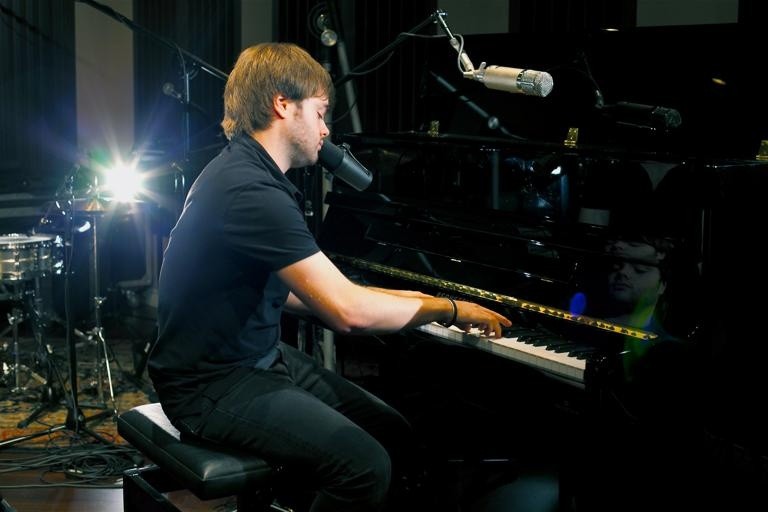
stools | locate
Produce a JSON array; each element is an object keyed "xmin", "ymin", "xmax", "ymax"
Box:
[{"xmin": 116, "ymin": 399, "xmax": 293, "ymax": 512}]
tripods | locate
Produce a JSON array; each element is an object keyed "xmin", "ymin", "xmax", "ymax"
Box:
[
  {"xmin": 0, "ymin": 215, "xmax": 118, "ymax": 448},
  {"xmin": 0, "ymin": 279, "xmax": 97, "ymax": 347},
  {"xmin": 13, "ymin": 343, "xmax": 107, "ymax": 429}
]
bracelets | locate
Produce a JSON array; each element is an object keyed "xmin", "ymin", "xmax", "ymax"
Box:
[{"xmin": 440, "ymin": 293, "xmax": 458, "ymax": 332}]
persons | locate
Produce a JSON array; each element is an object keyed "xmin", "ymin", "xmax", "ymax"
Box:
[
  {"xmin": 581, "ymin": 230, "xmax": 671, "ymax": 337},
  {"xmin": 145, "ymin": 38, "xmax": 513, "ymax": 510}
]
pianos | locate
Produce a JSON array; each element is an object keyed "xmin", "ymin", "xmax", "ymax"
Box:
[{"xmin": 307, "ymin": 23, "xmax": 761, "ymax": 510}]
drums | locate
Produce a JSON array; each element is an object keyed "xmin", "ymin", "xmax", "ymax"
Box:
[{"xmin": 1, "ymin": 233, "xmax": 63, "ymax": 283}]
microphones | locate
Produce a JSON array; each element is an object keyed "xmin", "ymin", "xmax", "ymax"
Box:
[
  {"xmin": 467, "ymin": 62, "xmax": 554, "ymax": 98},
  {"xmin": 162, "ymin": 83, "xmax": 210, "ymax": 119},
  {"xmin": 599, "ymin": 101, "xmax": 683, "ymax": 131},
  {"xmin": 318, "ymin": 140, "xmax": 374, "ymax": 193}
]
